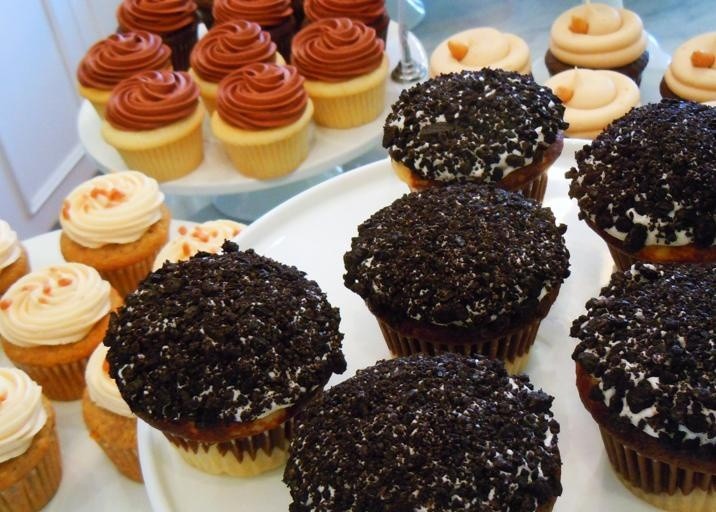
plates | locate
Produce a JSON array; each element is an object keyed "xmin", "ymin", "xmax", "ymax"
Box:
[
  {"xmin": 75, "ymin": 16, "xmax": 430, "ymax": 197},
  {"xmin": 136, "ymin": 138, "xmax": 667, "ymax": 512},
  {"xmin": 1, "ymin": 216, "xmax": 204, "ymax": 512}
]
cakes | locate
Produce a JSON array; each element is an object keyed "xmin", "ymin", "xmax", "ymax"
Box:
[
  {"xmin": 213, "ymin": 63, "xmax": 315, "ymax": 182},
  {"xmin": 284, "ymin": 351, "xmax": 561, "ymax": 512},
  {"xmin": 1, "ymin": 263, "xmax": 125, "ymax": 399},
  {"xmin": 116, "ymin": 0, "xmax": 208, "ymax": 69},
  {"xmin": 576, "ymin": 259, "xmax": 715, "ymax": 512},
  {"xmin": 344, "ymin": 183, "xmax": 572, "ymax": 374},
  {"xmin": 210, "ymin": 0, "xmax": 294, "ymax": 50},
  {"xmin": 1, "ymin": 219, "xmax": 32, "ymax": 295},
  {"xmin": 0, "ymin": 368, "xmax": 62, "ymax": 511},
  {"xmin": 153, "ymin": 219, "xmax": 246, "ymax": 275},
  {"xmin": 80, "ymin": 336, "xmax": 142, "ymax": 483},
  {"xmin": 544, "ymin": 69, "xmax": 639, "ymax": 139},
  {"xmin": 569, "ymin": 99, "xmax": 715, "ymax": 269},
  {"xmin": 190, "ymin": 19, "xmax": 285, "ymax": 116},
  {"xmin": 291, "ymin": 16, "xmax": 387, "ymax": 128},
  {"xmin": 77, "ymin": 29, "xmax": 174, "ymax": 120},
  {"xmin": 432, "ymin": 26, "xmax": 531, "ymax": 76},
  {"xmin": 108, "ymin": 246, "xmax": 340, "ymax": 475},
  {"xmin": 60, "ymin": 169, "xmax": 171, "ymax": 298},
  {"xmin": 102, "ymin": 70, "xmax": 203, "ymax": 180},
  {"xmin": 300, "ymin": 0, "xmax": 388, "ymax": 46},
  {"xmin": 548, "ymin": 6, "xmax": 650, "ymax": 82},
  {"xmin": 660, "ymin": 29, "xmax": 716, "ymax": 105},
  {"xmin": 383, "ymin": 69, "xmax": 566, "ymax": 209}
]
